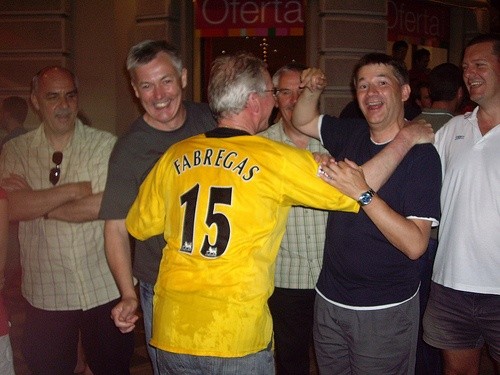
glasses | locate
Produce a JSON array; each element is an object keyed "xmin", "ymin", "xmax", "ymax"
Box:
[
  {"xmin": 259, "ymin": 85, "xmax": 280, "ymax": 97},
  {"xmin": 50, "ymin": 152, "xmax": 63, "ymax": 186}
]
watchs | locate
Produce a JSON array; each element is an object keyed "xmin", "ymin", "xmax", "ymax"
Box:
[{"xmin": 357, "ymin": 189, "xmax": 376, "ymax": 206}]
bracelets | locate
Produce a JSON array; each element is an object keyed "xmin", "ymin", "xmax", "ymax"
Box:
[{"xmin": 44, "ymin": 213, "xmax": 49, "ymax": 220}]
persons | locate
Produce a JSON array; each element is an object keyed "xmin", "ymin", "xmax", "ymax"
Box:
[{"xmin": 0, "ymin": 31, "xmax": 500, "ymax": 375}]
